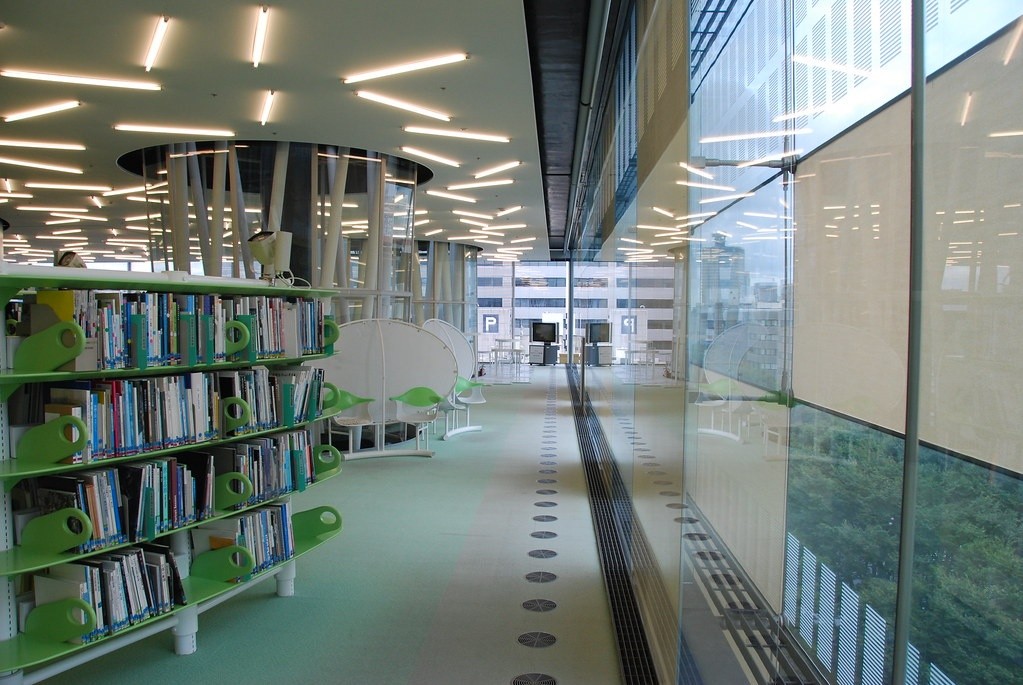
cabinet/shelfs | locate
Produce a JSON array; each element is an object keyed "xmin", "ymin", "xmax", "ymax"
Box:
[
  {"xmin": 585, "ymin": 345, "xmax": 612, "ymax": 367},
  {"xmin": 529, "ymin": 345, "xmax": 557, "ymax": 366},
  {"xmin": 0, "ymin": 262, "xmax": 343, "ymax": 685}
]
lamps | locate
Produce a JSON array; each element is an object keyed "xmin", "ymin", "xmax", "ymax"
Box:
[
  {"xmin": 339, "ymin": 50, "xmax": 471, "ymax": 85},
  {"xmin": 0, "ymin": 121, "xmax": 536, "ymax": 265},
  {"xmin": 58, "ymin": 252, "xmax": 87, "ymax": 268},
  {"xmin": 252, "ymin": 3, "xmax": 269, "ymax": 68},
  {"xmin": 248, "ymin": 230, "xmax": 295, "ymax": 286},
  {"xmin": 261, "ymin": 88, "xmax": 276, "ymax": 126},
  {"xmin": 144, "ymin": 10, "xmax": 171, "ymax": 72},
  {"xmin": 352, "ymin": 90, "xmax": 453, "ymax": 122},
  {"xmin": 0, "ymin": 65, "xmax": 163, "ymax": 91},
  {"xmin": 0, "ymin": 98, "xmax": 80, "ymax": 124}
]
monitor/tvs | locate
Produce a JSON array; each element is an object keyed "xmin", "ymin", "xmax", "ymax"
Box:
[
  {"xmin": 589, "ymin": 324, "xmax": 609, "ymax": 342},
  {"xmin": 532, "ymin": 322, "xmax": 556, "ymax": 342}
]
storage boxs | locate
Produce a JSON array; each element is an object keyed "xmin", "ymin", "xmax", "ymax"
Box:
[{"xmin": 31, "ymin": 304, "xmax": 98, "ymax": 372}]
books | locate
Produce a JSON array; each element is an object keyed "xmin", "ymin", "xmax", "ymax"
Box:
[
  {"xmin": 10, "ymin": 365, "xmax": 325, "ymax": 464},
  {"xmin": 15, "ymin": 428, "xmax": 329, "ymax": 554},
  {"xmin": 37, "ymin": 502, "xmax": 297, "ymax": 646},
  {"xmin": 36, "ymin": 286, "xmax": 325, "ymax": 373}
]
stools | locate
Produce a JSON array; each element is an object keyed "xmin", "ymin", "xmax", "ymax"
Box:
[{"xmin": 478, "ymin": 349, "xmax": 525, "ymax": 379}]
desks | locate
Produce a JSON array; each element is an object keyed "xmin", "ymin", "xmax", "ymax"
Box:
[
  {"xmin": 463, "ymin": 333, "xmax": 481, "ymax": 379},
  {"xmin": 492, "ymin": 339, "xmax": 525, "ymax": 379}
]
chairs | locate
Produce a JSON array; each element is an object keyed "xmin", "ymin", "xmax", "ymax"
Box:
[
  {"xmin": 325, "ymin": 390, "xmax": 375, "ymax": 454},
  {"xmin": 437, "ymin": 376, "xmax": 487, "ymax": 431},
  {"xmin": 389, "ymin": 386, "xmax": 445, "ymax": 451}
]
